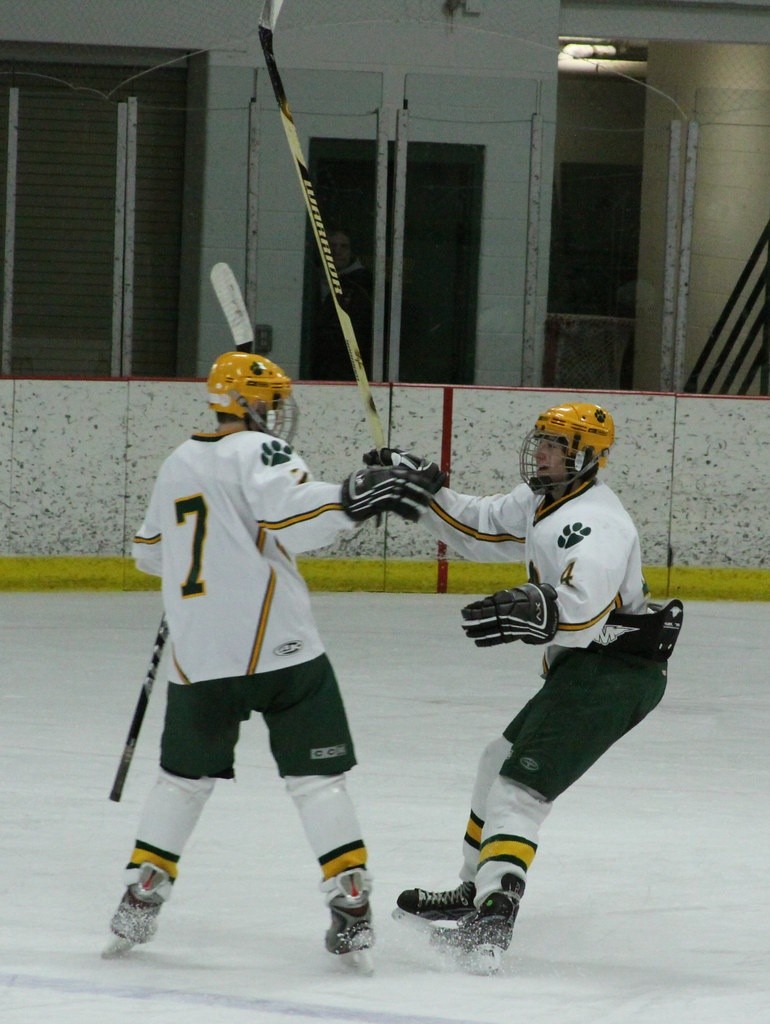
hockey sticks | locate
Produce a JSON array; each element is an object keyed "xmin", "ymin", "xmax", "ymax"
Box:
[
  {"xmin": 106, "ymin": 257, "xmax": 259, "ymax": 808},
  {"xmin": 255, "ymin": 0, "xmax": 395, "ymax": 469}
]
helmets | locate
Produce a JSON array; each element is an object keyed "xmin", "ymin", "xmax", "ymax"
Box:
[
  {"xmin": 519, "ymin": 403, "xmax": 615, "ymax": 495},
  {"xmin": 207, "ymin": 351, "xmax": 298, "ymax": 445}
]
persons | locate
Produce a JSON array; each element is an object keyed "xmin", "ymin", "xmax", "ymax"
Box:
[
  {"xmin": 312, "ymin": 225, "xmax": 373, "ymax": 382},
  {"xmin": 100, "ymin": 351, "xmax": 434, "ymax": 977},
  {"xmin": 363, "ymin": 403, "xmax": 668, "ymax": 973}
]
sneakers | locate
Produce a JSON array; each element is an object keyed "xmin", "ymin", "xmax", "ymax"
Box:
[
  {"xmin": 442, "ymin": 873, "xmax": 526, "ymax": 977},
  {"xmin": 391, "ymin": 881, "xmax": 479, "ymax": 952},
  {"xmin": 320, "ymin": 868, "xmax": 376, "ymax": 975},
  {"xmin": 102, "ymin": 862, "xmax": 169, "ymax": 960}
]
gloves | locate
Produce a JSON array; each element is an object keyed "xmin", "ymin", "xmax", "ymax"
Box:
[
  {"xmin": 342, "ymin": 466, "xmax": 433, "ymax": 524},
  {"xmin": 363, "ymin": 448, "xmax": 449, "ymax": 496},
  {"xmin": 461, "ymin": 584, "xmax": 559, "ymax": 648}
]
